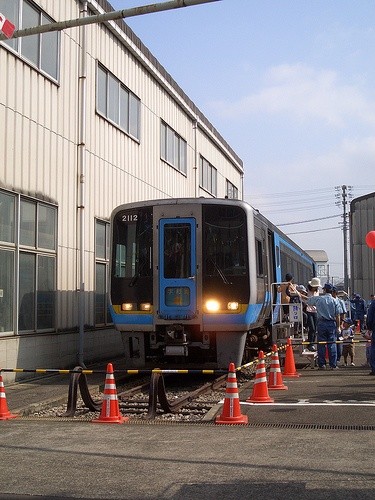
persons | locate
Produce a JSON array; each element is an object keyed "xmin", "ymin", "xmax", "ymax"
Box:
[
  {"xmin": 332, "ymin": 287, "xmax": 347, "ymax": 362},
  {"xmin": 305, "ymin": 278, "xmax": 322, "ymax": 350},
  {"xmin": 341, "ymin": 318, "xmax": 355, "ymax": 366},
  {"xmin": 350, "ymin": 294, "xmax": 367, "ymax": 331},
  {"xmin": 276, "ymin": 272, "xmax": 307, "ymax": 327},
  {"xmin": 368, "ymin": 294, "xmax": 375, "ymax": 305},
  {"xmin": 301, "ymin": 283, "xmax": 341, "ymax": 369},
  {"xmin": 365, "ymin": 300, "xmax": 375, "ymax": 375}
]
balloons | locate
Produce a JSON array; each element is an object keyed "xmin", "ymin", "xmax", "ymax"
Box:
[{"xmin": 366, "ymin": 231, "xmax": 375, "ymax": 248}]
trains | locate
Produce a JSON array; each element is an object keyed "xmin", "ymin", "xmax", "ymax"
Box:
[{"xmin": 108, "ymin": 196, "xmax": 316, "ymax": 369}]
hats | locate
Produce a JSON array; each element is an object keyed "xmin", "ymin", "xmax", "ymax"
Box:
[
  {"xmin": 322, "ymin": 283, "xmax": 333, "ymax": 290},
  {"xmin": 296, "ymin": 285, "xmax": 308, "ymax": 293},
  {"xmin": 341, "ymin": 318, "xmax": 353, "ymax": 324},
  {"xmin": 332, "ymin": 286, "xmax": 337, "ymax": 292},
  {"xmin": 356, "ymin": 295, "xmax": 361, "ymax": 299},
  {"xmin": 308, "ymin": 277, "xmax": 321, "ymax": 287},
  {"xmin": 286, "ymin": 273, "xmax": 294, "ymax": 282}
]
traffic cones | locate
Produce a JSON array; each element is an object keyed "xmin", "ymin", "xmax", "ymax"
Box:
[
  {"xmin": 215, "ymin": 363, "xmax": 248, "ymax": 424},
  {"xmin": 325, "ymin": 346, "xmax": 329, "ymax": 363},
  {"xmin": 267, "ymin": 345, "xmax": 288, "ymax": 390},
  {"xmin": 0, "ymin": 376, "xmax": 16, "ymax": 420},
  {"xmin": 281, "ymin": 338, "xmax": 301, "ymax": 377},
  {"xmin": 245, "ymin": 350, "xmax": 274, "ymax": 403},
  {"xmin": 355, "ymin": 320, "xmax": 360, "ymax": 333},
  {"xmin": 91, "ymin": 363, "xmax": 128, "ymax": 423}
]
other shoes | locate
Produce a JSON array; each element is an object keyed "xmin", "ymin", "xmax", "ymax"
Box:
[
  {"xmin": 351, "ymin": 362, "xmax": 355, "ymax": 366},
  {"xmin": 318, "ymin": 367, "xmax": 325, "ymax": 370},
  {"xmin": 306, "ymin": 345, "xmax": 316, "ymax": 351},
  {"xmin": 361, "ymin": 362, "xmax": 370, "ymax": 367},
  {"xmin": 294, "ymin": 334, "xmax": 302, "ymax": 338},
  {"xmin": 337, "ymin": 361, "xmax": 341, "ymax": 363},
  {"xmin": 342, "ymin": 363, "xmax": 348, "ymax": 366},
  {"xmin": 333, "ymin": 366, "xmax": 338, "ymax": 370},
  {"xmin": 369, "ymin": 371, "xmax": 375, "ymax": 376}
]
